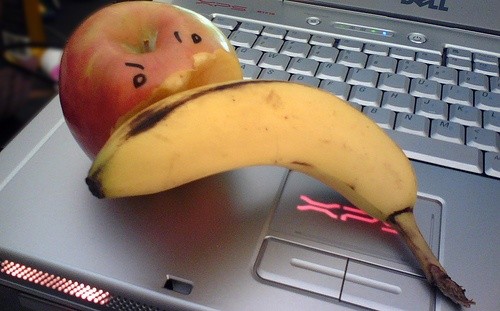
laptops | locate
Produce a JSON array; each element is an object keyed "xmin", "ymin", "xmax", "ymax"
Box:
[{"xmin": 1, "ymin": 0, "xmax": 500, "ymax": 309}]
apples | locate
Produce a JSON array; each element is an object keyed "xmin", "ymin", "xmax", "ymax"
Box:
[{"xmin": 59, "ymin": 0, "xmax": 243, "ymax": 163}]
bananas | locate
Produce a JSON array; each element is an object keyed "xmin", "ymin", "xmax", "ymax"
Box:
[{"xmin": 84, "ymin": 80, "xmax": 476, "ymax": 308}]
